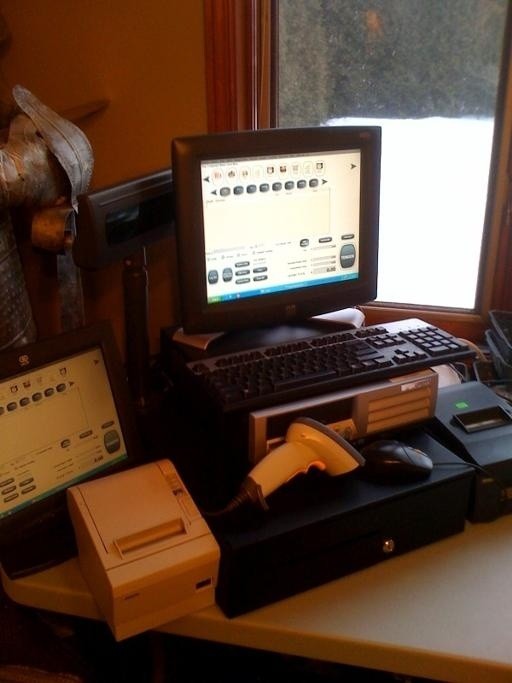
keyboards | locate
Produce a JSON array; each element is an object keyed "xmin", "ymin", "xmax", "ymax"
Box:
[{"xmin": 184, "ymin": 317, "xmax": 476, "ymax": 412}]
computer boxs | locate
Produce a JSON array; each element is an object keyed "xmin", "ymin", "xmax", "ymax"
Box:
[{"xmin": 159, "ymin": 320, "xmax": 439, "ymax": 471}]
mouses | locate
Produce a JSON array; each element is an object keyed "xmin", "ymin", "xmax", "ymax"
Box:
[{"xmin": 361, "ymin": 439, "xmax": 433, "ymax": 484}]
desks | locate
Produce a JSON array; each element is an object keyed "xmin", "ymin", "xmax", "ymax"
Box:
[{"xmin": 1, "ymin": 521, "xmax": 511, "ymax": 683}]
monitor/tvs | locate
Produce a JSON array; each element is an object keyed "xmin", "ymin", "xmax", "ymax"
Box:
[
  {"xmin": 172, "ymin": 127, "xmax": 381, "ymax": 350},
  {"xmin": 0, "ymin": 319, "xmax": 142, "ymax": 579}
]
